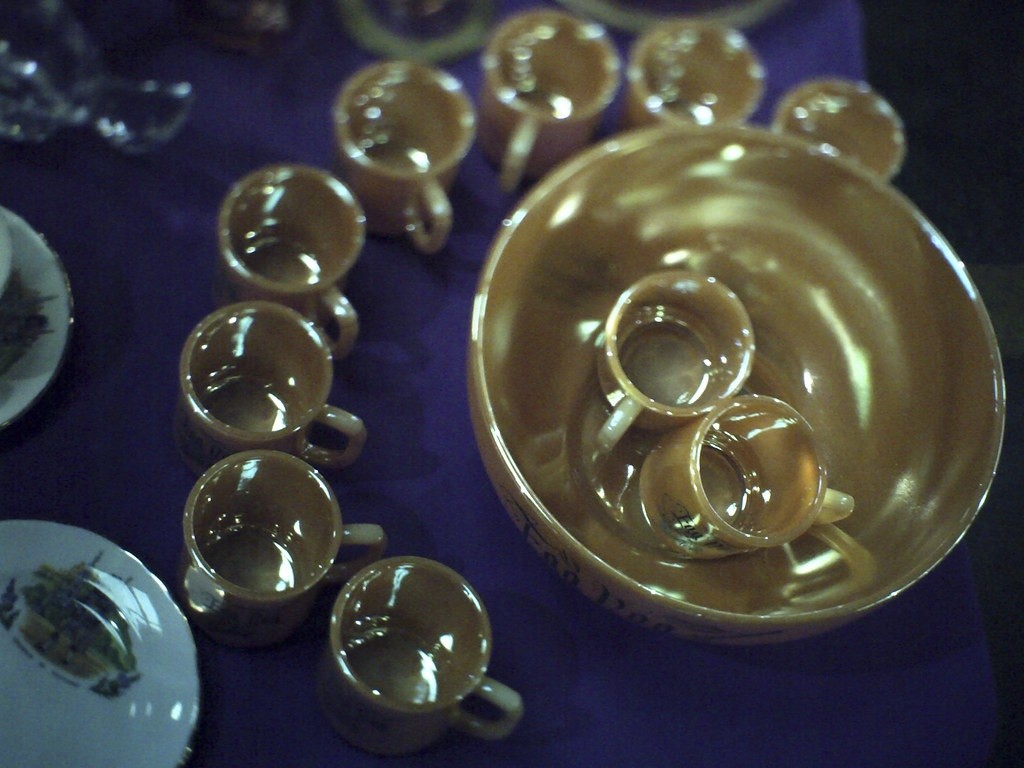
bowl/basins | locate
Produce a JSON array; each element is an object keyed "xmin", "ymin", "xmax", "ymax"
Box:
[{"xmin": 468, "ymin": 124, "xmax": 1008, "ymax": 636}]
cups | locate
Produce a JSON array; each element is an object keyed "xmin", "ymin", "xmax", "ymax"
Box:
[
  {"xmin": 772, "ymin": 78, "xmax": 908, "ymax": 187},
  {"xmin": 322, "ymin": 556, "xmax": 526, "ymax": 752},
  {"xmin": 202, "ymin": 162, "xmax": 368, "ymax": 357},
  {"xmin": 183, "ymin": 448, "xmax": 389, "ymax": 639},
  {"xmin": 329, "ymin": 61, "xmax": 482, "ymax": 253},
  {"xmin": 478, "ymin": 13, "xmax": 624, "ymax": 193},
  {"xmin": 176, "ymin": 298, "xmax": 370, "ymax": 475},
  {"xmin": 624, "ymin": 21, "xmax": 771, "ymax": 127},
  {"xmin": 593, "ymin": 272, "xmax": 757, "ymax": 454},
  {"xmin": 640, "ymin": 392, "xmax": 855, "ymax": 562}
]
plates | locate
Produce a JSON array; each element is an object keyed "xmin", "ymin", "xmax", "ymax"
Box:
[
  {"xmin": 338, "ymin": 1, "xmax": 498, "ymax": 63},
  {"xmin": 0, "ymin": 516, "xmax": 201, "ymax": 767},
  {"xmin": 0, "ymin": 208, "xmax": 71, "ymax": 428},
  {"xmin": 560, "ymin": 0, "xmax": 780, "ymax": 41}
]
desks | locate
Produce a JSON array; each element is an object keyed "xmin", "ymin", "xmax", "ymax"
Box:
[{"xmin": 0, "ymin": 0, "xmax": 999, "ymax": 768}]
